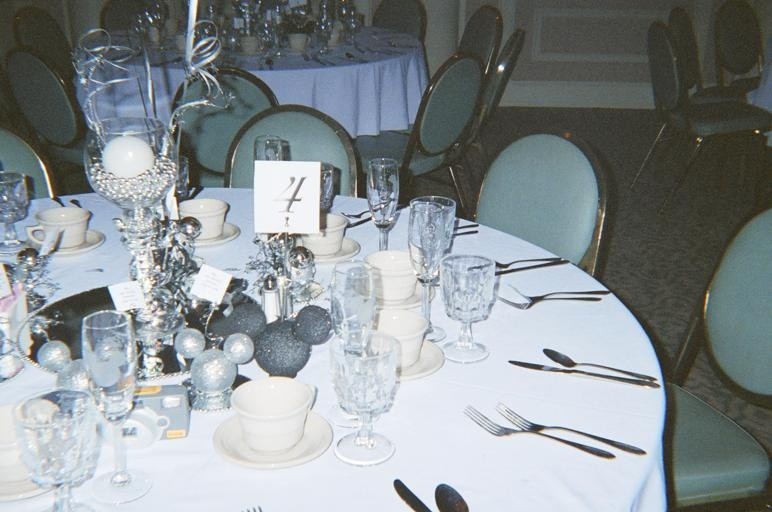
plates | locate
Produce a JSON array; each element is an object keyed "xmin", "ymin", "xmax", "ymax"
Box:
[
  {"xmin": 0, "ymin": 407, "xmax": 85, "ymax": 504},
  {"xmin": 33, "ymin": 230, "xmax": 104, "ymax": 257},
  {"xmin": 356, "ymin": 340, "xmax": 443, "ymax": 378},
  {"xmin": 314, "ymin": 235, "xmax": 361, "ymax": 260},
  {"xmin": 212, "ymin": 418, "xmax": 332, "ymax": 471},
  {"xmin": 191, "ymin": 223, "xmax": 242, "ymax": 249},
  {"xmin": 354, "ymin": 285, "xmax": 435, "ymax": 306}
]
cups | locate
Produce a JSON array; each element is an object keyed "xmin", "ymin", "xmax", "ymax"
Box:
[
  {"xmin": 299, "ymin": 213, "xmax": 349, "ymax": 254},
  {"xmin": 342, "ymin": 308, "xmax": 429, "ymax": 368},
  {"xmin": 251, "ymin": 136, "xmax": 292, "ymax": 160},
  {"xmin": 232, "ymin": 376, "xmax": 313, "ymax": 453},
  {"xmin": 176, "ymin": 199, "xmax": 230, "ymax": 239},
  {"xmin": 361, "ymin": 251, "xmax": 416, "ymax": 301},
  {"xmin": 25, "ymin": 209, "xmax": 89, "ymax": 247},
  {"xmin": 319, "ymin": 161, "xmax": 335, "ymax": 211}
]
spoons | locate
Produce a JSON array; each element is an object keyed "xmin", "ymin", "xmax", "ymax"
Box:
[
  {"xmin": 434, "ymin": 484, "xmax": 466, "ymax": 509},
  {"xmin": 543, "ymin": 345, "xmax": 654, "ymax": 384}
]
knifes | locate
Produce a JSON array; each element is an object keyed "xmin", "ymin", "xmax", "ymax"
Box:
[
  {"xmin": 507, "ymin": 357, "xmax": 660, "ymax": 393},
  {"xmin": 490, "ymin": 291, "xmax": 603, "ymax": 315},
  {"xmin": 392, "ymin": 477, "xmax": 434, "ymax": 510},
  {"xmin": 510, "ymin": 282, "xmax": 614, "ymax": 302}
]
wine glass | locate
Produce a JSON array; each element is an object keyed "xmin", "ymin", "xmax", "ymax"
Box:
[
  {"xmin": 411, "ymin": 196, "xmax": 458, "ymax": 303},
  {"xmin": 406, "ymin": 204, "xmax": 447, "ymax": 344},
  {"xmin": 367, "ymin": 158, "xmax": 401, "ymax": 252},
  {"xmin": 78, "ymin": 310, "xmax": 154, "ymax": 506},
  {"xmin": 18, "ymin": 388, "xmax": 107, "ymax": 510},
  {"xmin": 438, "ymin": 255, "xmax": 498, "ymax": 363},
  {"xmin": 120, "ymin": 1, "xmax": 381, "ymax": 64},
  {"xmin": 326, "ymin": 258, "xmax": 386, "ymax": 431},
  {"xmin": 1, "ymin": 170, "xmax": 30, "ymax": 253},
  {"xmin": 329, "ymin": 327, "xmax": 400, "ymax": 467}
]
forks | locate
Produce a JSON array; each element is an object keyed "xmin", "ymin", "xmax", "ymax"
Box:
[
  {"xmin": 460, "ymin": 405, "xmax": 621, "ymax": 460},
  {"xmin": 498, "ymin": 410, "xmax": 649, "ymax": 458}
]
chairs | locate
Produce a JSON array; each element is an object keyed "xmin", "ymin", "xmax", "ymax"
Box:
[
  {"xmin": 626, "ymin": 1, "xmax": 771, "ymax": 216},
  {"xmin": 351, "ymin": 0, "xmax": 526, "ymax": 221},
  {"xmin": 469, "ymin": 126, "xmax": 611, "ymax": 280},
  {"xmin": 99, "ymin": 0, "xmax": 169, "ymax": 36},
  {"xmin": 2, "ymin": 48, "xmax": 89, "ymax": 194},
  {"xmin": 663, "ymin": 201, "xmax": 772, "ymax": 512},
  {"xmin": 171, "ymin": 67, "xmax": 280, "ymax": 187},
  {"xmin": 0, "ymin": 118, "xmax": 58, "ymax": 201},
  {"xmin": 13, "ymin": 6, "xmax": 75, "ymax": 85},
  {"xmin": 223, "ymin": 104, "xmax": 364, "ymax": 198}
]
